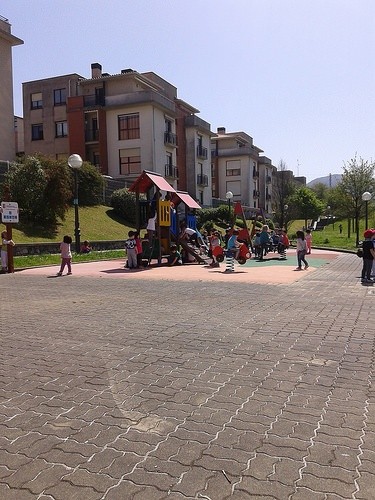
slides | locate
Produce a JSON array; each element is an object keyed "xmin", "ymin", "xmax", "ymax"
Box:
[{"xmin": 195, "ymin": 228, "xmax": 209, "ymax": 251}]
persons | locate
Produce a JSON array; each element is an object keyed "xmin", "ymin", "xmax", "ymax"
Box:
[
  {"xmin": 361, "ymin": 228, "xmax": 375, "ymax": 283},
  {"xmin": 292, "ymin": 231, "xmax": 309, "ymax": 271},
  {"xmin": 124, "ymin": 230, "xmax": 145, "ymax": 270},
  {"xmin": 146, "ymin": 209, "xmax": 158, "ymax": 252},
  {"xmin": 176, "ymin": 223, "xmax": 201, "ymax": 248},
  {"xmin": 227, "ymin": 229, "xmax": 244, "ymax": 260},
  {"xmin": 0, "ymin": 230, "xmax": 15, "ymax": 274},
  {"xmin": 253, "ymin": 225, "xmax": 290, "ymax": 256},
  {"xmin": 200, "ymin": 231, "xmax": 209, "ymax": 256},
  {"xmin": 305, "ymin": 230, "xmax": 312, "ymax": 254},
  {"xmin": 80, "ymin": 240, "xmax": 92, "ymax": 253},
  {"xmin": 167, "ymin": 244, "xmax": 182, "ymax": 266},
  {"xmin": 203, "ymin": 228, "xmax": 230, "ymax": 268},
  {"xmin": 150, "ymin": 192, "xmax": 176, "ymax": 220},
  {"xmin": 56, "ymin": 235, "xmax": 73, "ymax": 276}
]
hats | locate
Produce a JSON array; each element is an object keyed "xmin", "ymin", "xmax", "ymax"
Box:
[{"xmin": 364, "ymin": 229, "xmax": 375, "ymax": 237}]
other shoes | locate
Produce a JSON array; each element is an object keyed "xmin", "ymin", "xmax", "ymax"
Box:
[
  {"xmin": 199, "ymin": 247, "xmax": 204, "ymax": 253},
  {"xmin": 304, "ymin": 265, "xmax": 309, "ymax": 270},
  {"xmin": 129, "ymin": 267, "xmax": 132, "ymax": 270},
  {"xmin": 367, "ymin": 279, "xmax": 374, "ymax": 282},
  {"xmin": 56, "ymin": 273, "xmax": 62, "ymax": 276},
  {"xmin": 361, "ymin": 278, "xmax": 366, "ymax": 282},
  {"xmin": 67, "ymin": 272, "xmax": 72, "ymax": 275},
  {"xmin": 295, "ymin": 267, "xmax": 301, "ymax": 271},
  {"xmin": 134, "ymin": 267, "xmax": 138, "ymax": 269}
]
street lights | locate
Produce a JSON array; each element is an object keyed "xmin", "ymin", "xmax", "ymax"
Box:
[
  {"xmin": 67, "ymin": 154, "xmax": 84, "ymax": 254},
  {"xmin": 362, "ymin": 192, "xmax": 372, "ymax": 230},
  {"xmin": 226, "ymin": 191, "xmax": 234, "ymax": 214},
  {"xmin": 283, "ymin": 205, "xmax": 289, "ymax": 233},
  {"xmin": 326, "ymin": 205, "xmax": 331, "ymax": 226}
]
